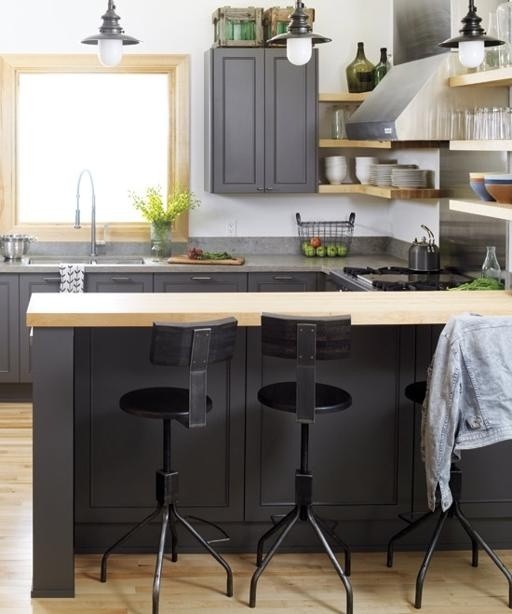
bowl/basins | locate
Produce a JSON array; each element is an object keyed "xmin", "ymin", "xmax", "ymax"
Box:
[
  {"xmin": 324, "ymin": 156, "xmax": 347, "ymax": 184},
  {"xmin": 0, "ymin": 234, "xmax": 32, "ymax": 259},
  {"xmin": 469, "ymin": 172, "xmax": 512, "ymax": 204},
  {"xmin": 354, "ymin": 157, "xmax": 378, "ymax": 184}
]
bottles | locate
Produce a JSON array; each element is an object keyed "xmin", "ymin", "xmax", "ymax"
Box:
[
  {"xmin": 345, "ymin": 42, "xmax": 392, "ymax": 92},
  {"xmin": 331, "ymin": 104, "xmax": 359, "ymax": 138},
  {"xmin": 476, "ymin": 2, "xmax": 512, "ymax": 72},
  {"xmin": 152, "ymin": 241, "xmax": 161, "ymax": 261},
  {"xmin": 481, "ymin": 246, "xmax": 501, "ymax": 283}
]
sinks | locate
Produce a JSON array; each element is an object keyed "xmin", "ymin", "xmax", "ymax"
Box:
[
  {"xmin": 93, "ymin": 253, "xmax": 147, "ymax": 268},
  {"xmin": 24, "ymin": 255, "xmax": 92, "ymax": 268}
]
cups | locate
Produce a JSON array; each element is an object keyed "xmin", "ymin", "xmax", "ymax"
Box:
[
  {"xmin": 450, "ymin": 111, "xmax": 463, "ymax": 140},
  {"xmin": 464, "ymin": 107, "xmax": 512, "ymax": 139}
]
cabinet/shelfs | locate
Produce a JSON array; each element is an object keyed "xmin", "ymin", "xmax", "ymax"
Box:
[
  {"xmin": 203, "ymin": 47, "xmax": 320, "ymax": 194},
  {"xmin": 448, "ymin": 66, "xmax": 512, "ymax": 221},
  {"xmin": 317, "ymin": 91, "xmax": 450, "ymax": 200},
  {"xmin": 153, "ymin": 272, "xmax": 322, "ymax": 292},
  {"xmin": 0, "ymin": 273, "xmax": 20, "ymax": 383},
  {"xmin": 20, "ymin": 273, "xmax": 153, "ymax": 383}
]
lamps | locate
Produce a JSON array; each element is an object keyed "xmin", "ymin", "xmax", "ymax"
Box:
[
  {"xmin": 438, "ymin": 0, "xmax": 505, "ymax": 69},
  {"xmin": 266, "ymin": 0, "xmax": 332, "ymax": 66},
  {"xmin": 81, "ymin": 0, "xmax": 139, "ymax": 68}
]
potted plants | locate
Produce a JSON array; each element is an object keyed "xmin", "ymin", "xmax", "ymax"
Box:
[{"xmin": 127, "ymin": 184, "xmax": 202, "ymax": 260}]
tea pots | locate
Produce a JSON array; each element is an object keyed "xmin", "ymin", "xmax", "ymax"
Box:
[{"xmin": 408, "ymin": 223, "xmax": 440, "ymax": 271}]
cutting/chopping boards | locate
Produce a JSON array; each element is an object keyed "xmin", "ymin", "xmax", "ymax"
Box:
[{"xmin": 168, "ymin": 254, "xmax": 244, "ymax": 265}]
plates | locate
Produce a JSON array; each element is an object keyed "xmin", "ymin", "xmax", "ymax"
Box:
[
  {"xmin": 391, "ymin": 169, "xmax": 427, "ymax": 188},
  {"xmin": 371, "ymin": 164, "xmax": 416, "ymax": 185}
]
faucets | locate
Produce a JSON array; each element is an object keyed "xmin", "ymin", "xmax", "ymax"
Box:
[{"xmin": 69, "ymin": 166, "xmax": 97, "ymax": 258}]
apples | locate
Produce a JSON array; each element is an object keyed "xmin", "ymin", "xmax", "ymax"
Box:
[{"xmin": 303, "ymin": 236, "xmax": 349, "ymax": 258}]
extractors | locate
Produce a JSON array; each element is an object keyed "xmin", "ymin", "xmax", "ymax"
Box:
[{"xmin": 344, "ymin": 51, "xmax": 469, "ymax": 141}]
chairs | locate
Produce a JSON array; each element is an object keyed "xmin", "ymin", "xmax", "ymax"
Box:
[
  {"xmin": 100, "ymin": 317, "xmax": 239, "ymax": 614},
  {"xmin": 248, "ymin": 313, "xmax": 354, "ymax": 613},
  {"xmin": 386, "ymin": 315, "xmax": 512, "ymax": 609}
]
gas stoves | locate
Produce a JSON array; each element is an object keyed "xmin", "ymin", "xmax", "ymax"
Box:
[{"xmin": 331, "ymin": 265, "xmax": 474, "ymax": 291}]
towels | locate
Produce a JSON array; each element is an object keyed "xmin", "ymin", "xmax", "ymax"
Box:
[{"xmin": 59, "ymin": 263, "xmax": 86, "ymax": 294}]
